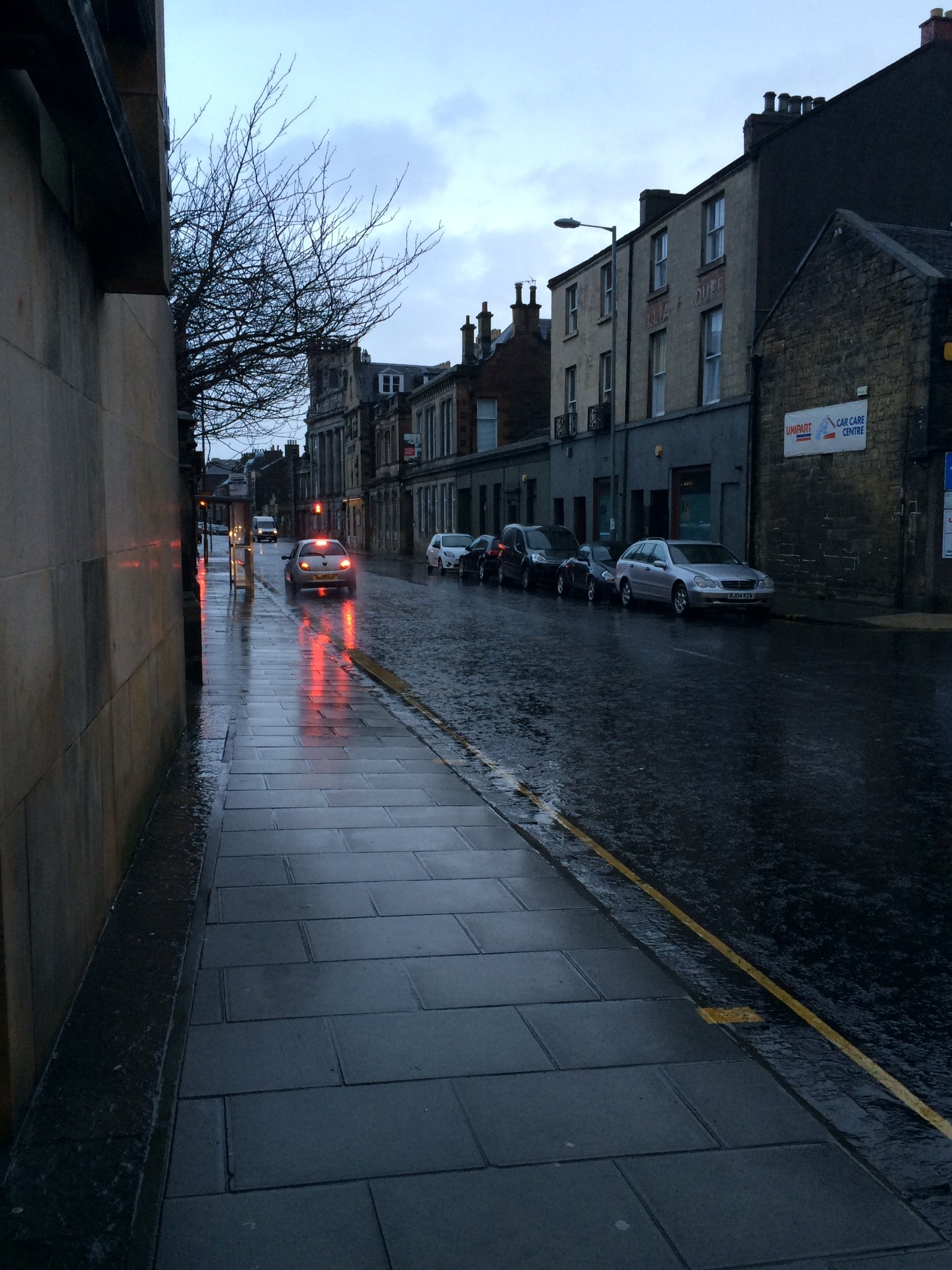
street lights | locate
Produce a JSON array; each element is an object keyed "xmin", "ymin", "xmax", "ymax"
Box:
[
  {"xmin": 199, "ymin": 501, "xmax": 208, "ymax": 563},
  {"xmin": 553, "ymin": 217, "xmax": 619, "ymax": 544}
]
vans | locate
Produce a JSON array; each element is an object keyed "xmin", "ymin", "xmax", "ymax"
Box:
[{"xmin": 252, "ymin": 516, "xmax": 278, "ymax": 543}]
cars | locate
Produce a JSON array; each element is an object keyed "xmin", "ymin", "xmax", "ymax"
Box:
[
  {"xmin": 427, "ymin": 522, "xmax": 776, "ymax": 616},
  {"xmin": 197, "ymin": 522, "xmax": 229, "ymax": 535},
  {"xmin": 282, "ymin": 539, "xmax": 357, "ymax": 593},
  {"xmin": 197, "ymin": 526, "xmax": 202, "ymax": 542}
]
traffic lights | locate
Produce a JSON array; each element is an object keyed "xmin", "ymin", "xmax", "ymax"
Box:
[{"xmin": 314, "ymin": 505, "xmax": 322, "ymax": 516}]
